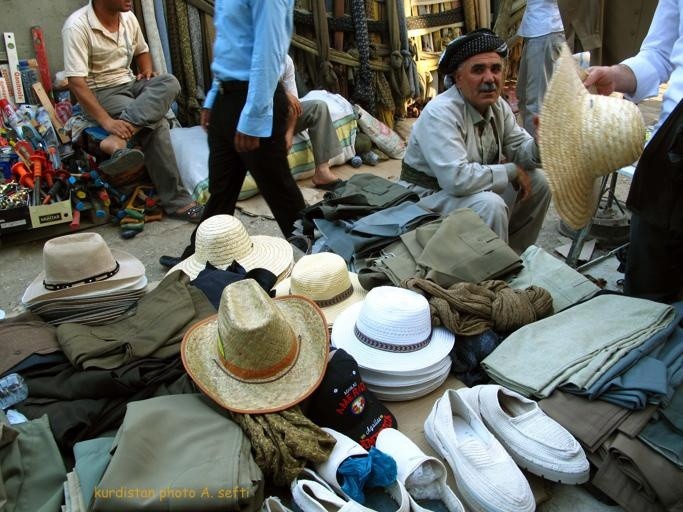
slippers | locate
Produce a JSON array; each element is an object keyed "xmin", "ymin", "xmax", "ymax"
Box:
[
  {"xmin": 316, "ymin": 178, "xmax": 343, "ymax": 190},
  {"xmin": 170, "ymin": 202, "xmax": 205, "ymax": 223},
  {"xmin": 98, "ymin": 149, "xmax": 144, "ymax": 175}
]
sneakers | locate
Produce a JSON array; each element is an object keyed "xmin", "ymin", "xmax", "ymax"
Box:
[{"xmin": 260, "ymin": 384, "xmax": 590, "ymax": 512}]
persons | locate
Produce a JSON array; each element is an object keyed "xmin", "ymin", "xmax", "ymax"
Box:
[
  {"xmin": 396, "ymin": 26, "xmax": 551, "ymax": 255},
  {"xmin": 158, "ymin": 1, "xmax": 307, "ymax": 270},
  {"xmin": 581, "ymin": 0, "xmax": 682, "ymax": 305},
  {"xmin": 492, "ymin": 1, "xmax": 602, "ymax": 144},
  {"xmin": 61, "ymin": 1, "xmax": 205, "ymax": 224},
  {"xmin": 278, "ymin": 53, "xmax": 344, "ymax": 190}
]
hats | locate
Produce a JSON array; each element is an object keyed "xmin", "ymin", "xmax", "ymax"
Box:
[
  {"xmin": 22, "ymin": 232, "xmax": 148, "ymax": 326},
  {"xmin": 438, "ymin": 28, "xmax": 507, "ymax": 74},
  {"xmin": 167, "ymin": 214, "xmax": 456, "ymax": 451},
  {"xmin": 538, "ymin": 42, "xmax": 646, "ymax": 230}
]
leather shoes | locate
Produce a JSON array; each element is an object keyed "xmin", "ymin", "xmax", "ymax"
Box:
[{"xmin": 160, "ymin": 256, "xmax": 180, "ymax": 267}]
